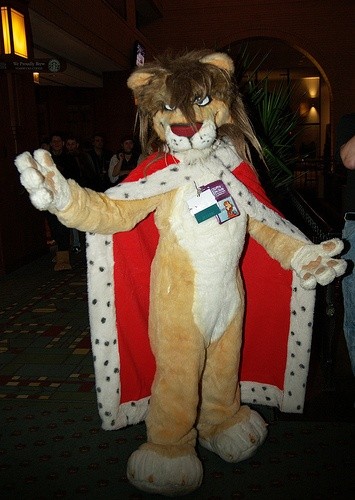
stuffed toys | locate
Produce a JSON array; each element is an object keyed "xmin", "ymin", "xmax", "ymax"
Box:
[{"xmin": 13, "ymin": 49, "xmax": 349, "ymax": 499}]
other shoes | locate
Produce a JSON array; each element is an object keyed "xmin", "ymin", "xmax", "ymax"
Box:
[
  {"xmin": 54, "ymin": 250, "xmax": 73, "ymax": 271},
  {"xmin": 72, "ymin": 246, "xmax": 82, "ymax": 257}
]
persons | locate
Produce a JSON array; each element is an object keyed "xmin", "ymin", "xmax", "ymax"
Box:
[
  {"xmin": 330, "ymin": 114, "xmax": 355, "ymax": 384},
  {"xmin": 29, "ymin": 132, "xmax": 141, "ymax": 273}
]
